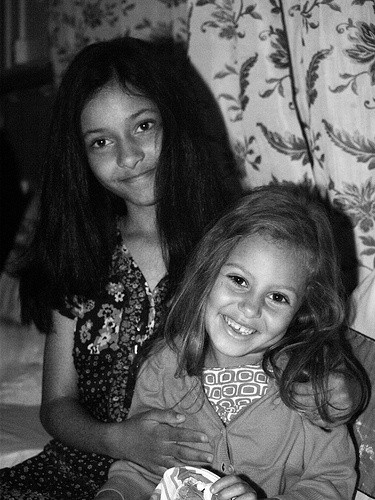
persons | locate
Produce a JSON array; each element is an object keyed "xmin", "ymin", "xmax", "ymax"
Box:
[
  {"xmin": 95, "ymin": 183, "xmax": 370, "ymax": 500},
  {"xmin": 0, "ymin": 36, "xmax": 246, "ymax": 500}
]
